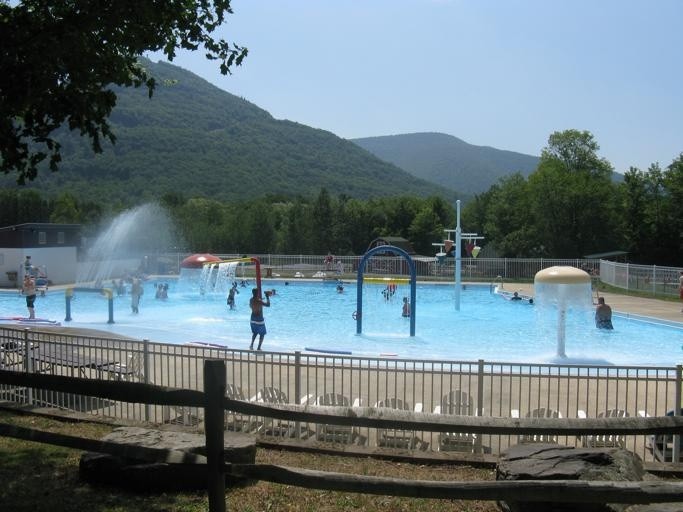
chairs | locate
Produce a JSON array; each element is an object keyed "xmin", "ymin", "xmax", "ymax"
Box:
[{"xmin": 115, "ymin": 351, "xmax": 142, "ymax": 382}]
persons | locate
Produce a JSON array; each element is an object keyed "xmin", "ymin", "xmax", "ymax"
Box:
[
  {"xmin": 229, "ymin": 282, "xmax": 240, "ymax": 310},
  {"xmin": 402, "ymin": 297, "xmax": 410, "ymax": 317},
  {"xmin": 270, "ymin": 290, "xmax": 277, "ymax": 296},
  {"xmin": 381, "ymin": 284, "xmax": 398, "ymax": 300},
  {"xmin": 95, "ymin": 265, "xmax": 170, "ymax": 313},
  {"xmin": 579, "ymin": 264, "xmax": 599, "ymax": 276},
  {"xmin": 595, "ymin": 296, "xmax": 614, "ymax": 330},
  {"xmin": 23, "ymin": 255, "xmax": 32, "ymax": 280},
  {"xmin": 336, "ymin": 283, "xmax": 344, "ymax": 293},
  {"xmin": 21, "ymin": 276, "xmax": 37, "ymax": 319},
  {"xmin": 249, "ymin": 288, "xmax": 270, "ymax": 351},
  {"xmin": 678, "ymin": 272, "xmax": 683, "ymax": 313},
  {"xmin": 509, "ymin": 292, "xmax": 522, "ymax": 301},
  {"xmin": 240, "ymin": 280, "xmax": 249, "ymax": 289}
]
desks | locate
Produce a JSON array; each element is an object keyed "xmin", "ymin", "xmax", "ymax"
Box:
[
  {"xmin": 18, "ymin": 352, "xmax": 119, "ymax": 379},
  {"xmin": 0, "ymin": 342, "xmax": 40, "ymax": 367}
]
